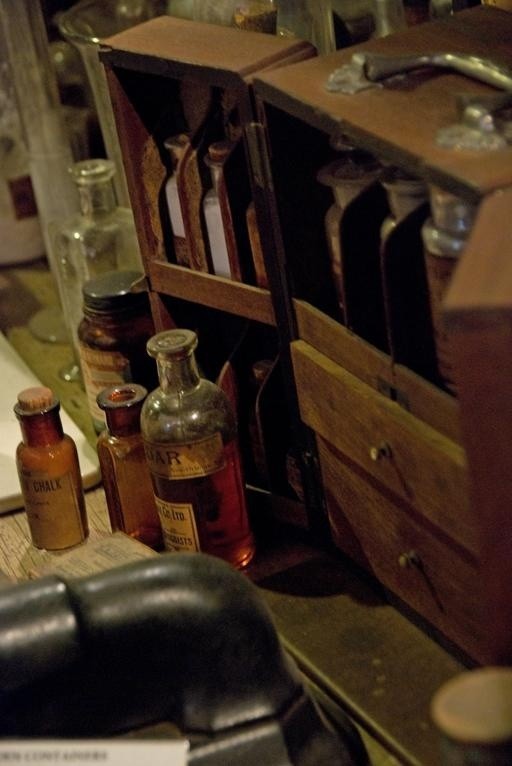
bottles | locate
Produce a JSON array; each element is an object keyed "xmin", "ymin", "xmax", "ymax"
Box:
[
  {"xmin": 71, "ymin": 271, "xmax": 154, "ymax": 437},
  {"xmin": 163, "ymin": 134, "xmax": 270, "ymax": 295},
  {"xmin": 89, "ymin": 383, "xmax": 160, "ymax": 548},
  {"xmin": 138, "ymin": 329, "xmax": 257, "ymax": 572},
  {"xmin": 319, "ymin": 161, "xmax": 472, "ymax": 390},
  {"xmin": 250, "ymin": 359, "xmax": 272, "ymax": 456},
  {"xmin": 50, "ymin": 159, "xmax": 156, "ymax": 388},
  {"xmin": 12, "ymin": 388, "xmax": 88, "ymax": 550}
]
